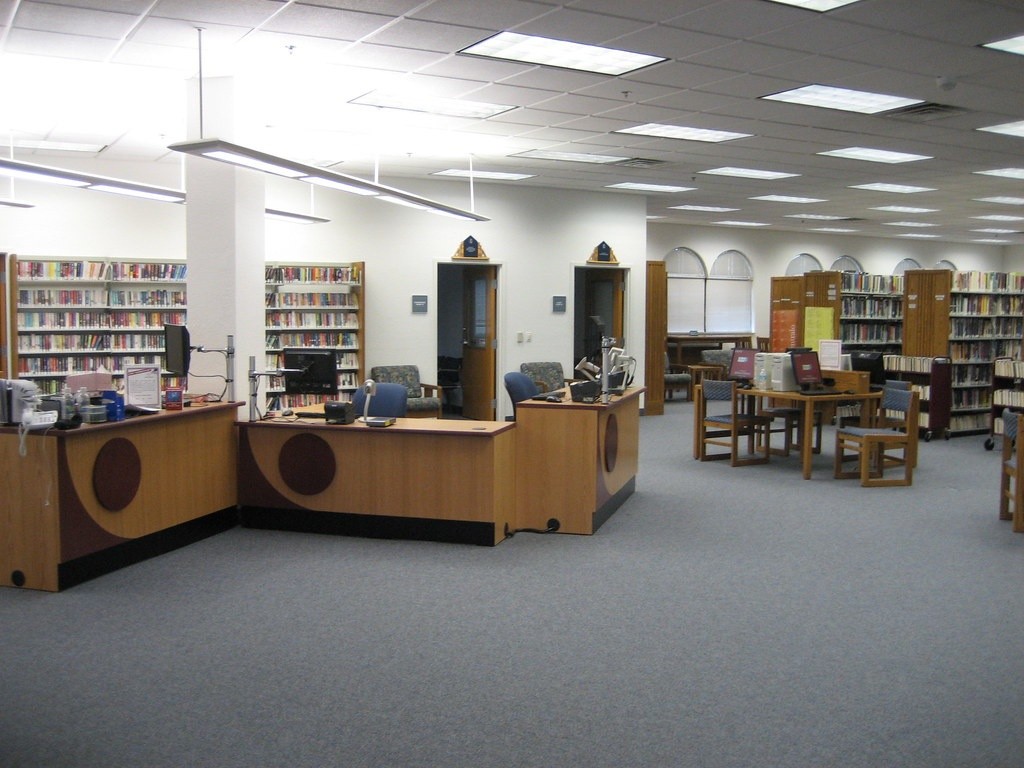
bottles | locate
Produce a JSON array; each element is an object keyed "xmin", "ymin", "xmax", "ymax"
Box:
[
  {"xmin": 759, "ymin": 369, "xmax": 767, "ymax": 392},
  {"xmin": 74, "ymin": 386, "xmax": 90, "ymax": 425},
  {"xmin": 61, "ymin": 383, "xmax": 75, "ymax": 419}
]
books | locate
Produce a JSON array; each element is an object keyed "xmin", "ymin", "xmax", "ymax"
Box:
[
  {"xmin": 881, "ymin": 355, "xmax": 934, "ymax": 429},
  {"xmin": 15, "ymin": 260, "xmax": 188, "ymax": 395},
  {"xmin": 949, "ymin": 270, "xmax": 1024, "ymax": 435},
  {"xmin": 835, "ymin": 271, "xmax": 904, "ymax": 417},
  {"xmin": 266, "ymin": 265, "xmax": 360, "ymax": 409}
]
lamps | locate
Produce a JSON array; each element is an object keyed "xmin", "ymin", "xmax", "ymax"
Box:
[{"xmin": 0, "ymin": 28, "xmax": 493, "ymax": 223}]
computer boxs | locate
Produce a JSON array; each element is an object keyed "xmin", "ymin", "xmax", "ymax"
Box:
[{"xmin": 753, "ymin": 352, "xmax": 802, "ymax": 392}]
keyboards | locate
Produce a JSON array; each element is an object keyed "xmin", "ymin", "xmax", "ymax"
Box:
[
  {"xmin": 295, "ymin": 412, "xmax": 326, "ymax": 418},
  {"xmin": 532, "ymin": 392, "xmax": 566, "ymax": 401},
  {"xmin": 800, "ymin": 390, "xmax": 842, "ymax": 396}
]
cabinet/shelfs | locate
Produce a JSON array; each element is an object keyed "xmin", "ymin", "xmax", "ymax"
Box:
[
  {"xmin": 803, "ymin": 266, "xmax": 1024, "ymax": 450},
  {"xmin": 0, "ymin": 392, "xmax": 246, "ymax": 592},
  {"xmin": 643, "ymin": 260, "xmax": 668, "ymax": 417},
  {"xmin": 7, "ymin": 254, "xmax": 365, "ymax": 410},
  {"xmin": 517, "ymin": 383, "xmax": 646, "ymax": 535},
  {"xmin": 235, "ymin": 414, "xmax": 517, "ymax": 547}
]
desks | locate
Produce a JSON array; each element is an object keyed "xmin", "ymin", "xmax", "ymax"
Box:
[
  {"xmin": 737, "ymin": 386, "xmax": 884, "ymax": 480},
  {"xmin": 687, "ymin": 365, "xmax": 724, "ymax": 401}
]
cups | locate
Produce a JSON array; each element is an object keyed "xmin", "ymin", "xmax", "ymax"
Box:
[
  {"xmin": 101, "ymin": 389, "xmax": 124, "ymax": 421},
  {"xmin": 89, "ymin": 396, "xmax": 102, "ymax": 405}
]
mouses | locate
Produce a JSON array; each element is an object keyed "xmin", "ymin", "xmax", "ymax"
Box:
[
  {"xmin": 546, "ymin": 396, "xmax": 562, "ymax": 403},
  {"xmin": 843, "ymin": 390, "xmax": 855, "ymax": 394},
  {"xmin": 744, "ymin": 385, "xmax": 751, "ymax": 390},
  {"xmin": 282, "ymin": 410, "xmax": 293, "ymax": 416}
]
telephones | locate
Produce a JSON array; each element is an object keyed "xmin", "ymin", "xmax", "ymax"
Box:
[{"xmin": 22, "ymin": 408, "xmax": 58, "ymax": 430}]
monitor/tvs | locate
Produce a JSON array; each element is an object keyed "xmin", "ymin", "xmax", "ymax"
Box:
[
  {"xmin": 728, "ymin": 348, "xmax": 762, "ymax": 388},
  {"xmin": 785, "ymin": 347, "xmax": 812, "ymax": 354},
  {"xmin": 584, "ymin": 315, "xmax": 616, "ymax": 404},
  {"xmin": 283, "ymin": 348, "xmax": 338, "ymax": 396},
  {"xmin": 164, "ymin": 322, "xmax": 191, "ymax": 375},
  {"xmin": 791, "ymin": 352, "xmax": 821, "ymax": 391},
  {"xmin": 850, "ymin": 350, "xmax": 886, "ymax": 393}
]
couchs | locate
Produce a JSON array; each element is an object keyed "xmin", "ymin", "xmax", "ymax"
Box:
[
  {"xmin": 371, "ymin": 365, "xmax": 443, "ymax": 420},
  {"xmin": 519, "ymin": 362, "xmax": 584, "ymax": 402},
  {"xmin": 664, "ymin": 351, "xmax": 692, "ymax": 402}
]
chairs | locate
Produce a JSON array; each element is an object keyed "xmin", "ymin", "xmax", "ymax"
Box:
[
  {"xmin": 352, "ymin": 381, "xmax": 407, "ymax": 418},
  {"xmin": 1000, "ymin": 408, "xmax": 1024, "ymax": 533},
  {"xmin": 505, "ymin": 372, "xmax": 540, "ymax": 420},
  {"xmin": 735, "ymin": 336, "xmax": 752, "ymax": 348},
  {"xmin": 757, "ymin": 336, "xmax": 770, "ymax": 353},
  {"xmin": 694, "ymin": 363, "xmax": 922, "ymax": 487}
]
